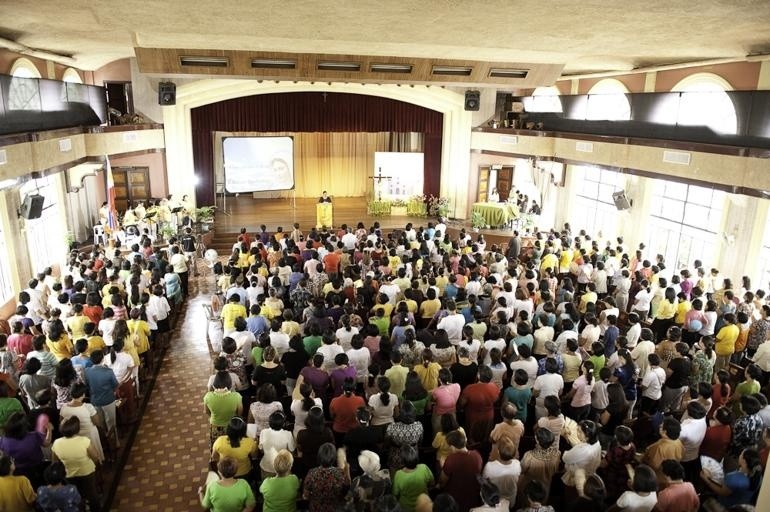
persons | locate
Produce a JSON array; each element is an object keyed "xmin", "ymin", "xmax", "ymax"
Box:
[{"xmin": 0, "ymin": 184, "xmax": 770, "ymax": 511}]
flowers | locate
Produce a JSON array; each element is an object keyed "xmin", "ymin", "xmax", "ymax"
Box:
[{"xmin": 422, "ymin": 194, "xmax": 448, "ymax": 215}]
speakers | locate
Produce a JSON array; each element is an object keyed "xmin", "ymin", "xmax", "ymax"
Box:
[
  {"xmin": 158, "ymin": 81, "xmax": 176, "ymax": 106},
  {"xmin": 612, "ymin": 190, "xmax": 631, "ymax": 211},
  {"xmin": 464, "ymin": 90, "xmax": 480, "ymax": 112},
  {"xmin": 19, "ymin": 194, "xmax": 44, "ymax": 219}
]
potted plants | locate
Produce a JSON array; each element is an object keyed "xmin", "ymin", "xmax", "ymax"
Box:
[
  {"xmin": 472, "ymin": 213, "xmax": 486, "ymax": 232},
  {"xmin": 438, "ymin": 205, "xmax": 450, "ymax": 223}
]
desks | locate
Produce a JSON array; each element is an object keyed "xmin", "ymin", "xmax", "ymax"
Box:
[{"xmin": 473, "ymin": 202, "xmax": 520, "ymax": 229}]
